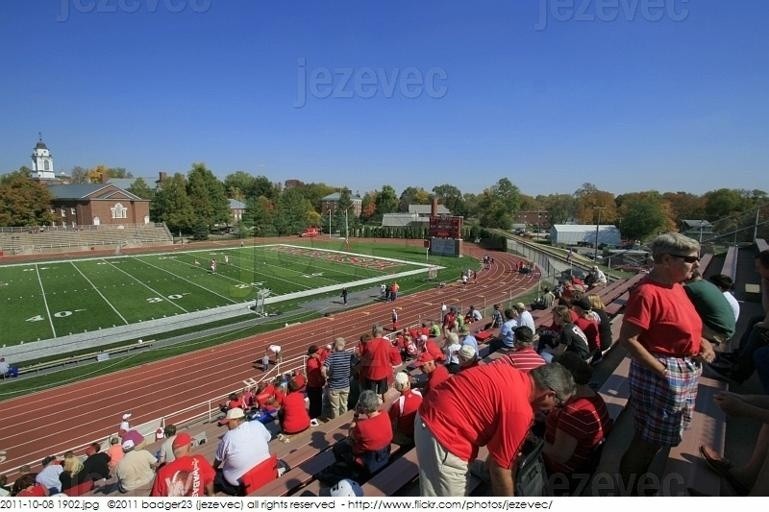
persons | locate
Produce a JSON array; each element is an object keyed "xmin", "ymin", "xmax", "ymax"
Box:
[{"xmin": 1, "ymin": 233, "xmax": 768, "ymax": 497}]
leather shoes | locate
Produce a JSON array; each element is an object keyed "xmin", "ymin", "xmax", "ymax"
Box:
[{"xmin": 700, "ymin": 444, "xmax": 751, "ymax": 496}]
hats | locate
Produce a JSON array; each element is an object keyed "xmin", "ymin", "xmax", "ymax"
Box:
[
  {"xmin": 172, "ymin": 434, "xmax": 196, "ymax": 449},
  {"xmin": 573, "ymin": 297, "xmax": 591, "ymax": 311},
  {"xmin": 220, "ymin": 407, "xmax": 244, "ymax": 424},
  {"xmin": 458, "ymin": 345, "xmax": 475, "ymax": 359},
  {"xmin": 552, "ymin": 351, "xmax": 595, "ymax": 384},
  {"xmin": 123, "ymin": 440, "xmax": 135, "ymax": 450},
  {"xmin": 123, "ymin": 413, "xmax": 131, "ymax": 420},
  {"xmin": 512, "ymin": 326, "xmax": 532, "ymax": 343},
  {"xmin": 415, "ymin": 352, "xmax": 433, "ymax": 368}
]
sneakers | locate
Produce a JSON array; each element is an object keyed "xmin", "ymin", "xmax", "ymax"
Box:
[{"xmin": 702, "ymin": 350, "xmax": 743, "ymax": 385}]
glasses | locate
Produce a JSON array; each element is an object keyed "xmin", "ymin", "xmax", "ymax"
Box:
[{"xmin": 670, "ymin": 253, "xmax": 700, "ymax": 262}]
